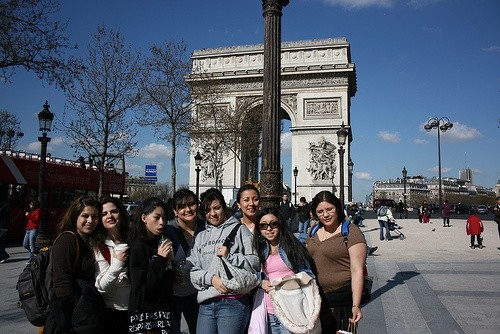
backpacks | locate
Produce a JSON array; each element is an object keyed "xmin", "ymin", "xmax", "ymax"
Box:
[
  {"xmin": 309, "ymin": 221, "xmax": 373, "ymax": 305},
  {"xmin": 376, "ymin": 206, "xmax": 389, "ymax": 216},
  {"xmin": 18, "ymin": 231, "xmax": 80, "ymax": 327}
]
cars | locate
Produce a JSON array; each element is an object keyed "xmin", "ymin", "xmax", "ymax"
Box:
[{"xmin": 425, "ymin": 203, "xmax": 489, "ymax": 215}]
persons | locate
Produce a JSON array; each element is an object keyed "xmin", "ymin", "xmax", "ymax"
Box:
[
  {"xmin": 306, "ymin": 191, "xmax": 368, "ymax": 334},
  {"xmin": 127, "ymin": 197, "xmax": 173, "ymax": 334},
  {"xmin": 90, "ymin": 197, "xmax": 131, "ymax": 334},
  {"xmin": 254, "ymin": 207, "xmax": 315, "ymax": 334},
  {"xmin": 0, "ymin": 192, "xmax": 10, "ymax": 263},
  {"xmin": 43, "ymin": 196, "xmax": 101, "ymax": 334},
  {"xmin": 280, "ymin": 194, "xmax": 319, "ymax": 243},
  {"xmin": 377, "ymin": 203, "xmax": 403, "ymax": 241},
  {"xmin": 441, "ymin": 199, "xmax": 450, "ymax": 227},
  {"xmin": 232, "ymin": 202, "xmax": 242, "ymax": 218},
  {"xmin": 186, "ymin": 188, "xmax": 260, "ymax": 334},
  {"xmin": 203, "ymin": 149, "xmax": 217, "ymax": 177},
  {"xmin": 494, "ymin": 196, "xmax": 500, "ymax": 249},
  {"xmin": 343, "ymin": 202, "xmax": 365, "ymax": 227},
  {"xmin": 23, "ymin": 200, "xmax": 41, "ymax": 264},
  {"xmin": 466, "ymin": 211, "xmax": 484, "ymax": 249},
  {"xmin": 394, "ymin": 200, "xmax": 408, "ymax": 219},
  {"xmin": 237, "ymin": 184, "xmax": 261, "ymax": 234},
  {"xmin": 167, "ymin": 189, "xmax": 204, "ymax": 334},
  {"xmin": 417, "ymin": 206, "xmax": 430, "ymax": 222},
  {"xmin": 310, "ymin": 143, "xmax": 335, "ymax": 179}
]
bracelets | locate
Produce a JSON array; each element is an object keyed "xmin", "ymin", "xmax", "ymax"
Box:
[{"xmin": 353, "ymin": 305, "xmax": 361, "ymax": 308}]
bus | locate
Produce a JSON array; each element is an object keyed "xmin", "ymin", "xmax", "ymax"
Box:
[{"xmin": 0, "ymin": 148, "xmax": 126, "ymax": 246}]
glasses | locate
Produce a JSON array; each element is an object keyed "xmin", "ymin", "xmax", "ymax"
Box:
[
  {"xmin": 257, "ymin": 221, "xmax": 279, "ymax": 230},
  {"xmin": 315, "ymin": 206, "xmax": 335, "ymax": 215}
]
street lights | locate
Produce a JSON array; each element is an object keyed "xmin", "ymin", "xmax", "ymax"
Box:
[
  {"xmin": 194, "ymin": 150, "xmax": 202, "ymax": 197},
  {"xmin": 35, "ymin": 100, "xmax": 51, "ymax": 252},
  {"xmin": 336, "ymin": 121, "xmax": 349, "ymax": 208},
  {"xmin": 402, "ymin": 166, "xmax": 408, "ymax": 218},
  {"xmin": 293, "ymin": 166, "xmax": 298, "ymax": 206},
  {"xmin": 424, "ymin": 116, "xmax": 453, "ymax": 217}
]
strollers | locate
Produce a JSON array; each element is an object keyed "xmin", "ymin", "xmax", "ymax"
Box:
[{"xmin": 388, "ymin": 222, "xmax": 404, "ymax": 240}]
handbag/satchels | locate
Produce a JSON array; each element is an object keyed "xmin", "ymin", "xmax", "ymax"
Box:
[
  {"xmin": 335, "ymin": 320, "xmax": 360, "ymax": 334},
  {"xmin": 218, "ymin": 224, "xmax": 260, "ymax": 291}
]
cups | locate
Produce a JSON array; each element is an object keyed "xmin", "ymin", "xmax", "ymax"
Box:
[
  {"xmin": 158, "ymin": 237, "xmax": 174, "ymax": 261},
  {"xmin": 114, "ymin": 243, "xmax": 127, "ymax": 255}
]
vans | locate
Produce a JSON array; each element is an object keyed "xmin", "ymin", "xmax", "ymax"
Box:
[{"xmin": 373, "ymin": 198, "xmax": 396, "ymax": 212}]
